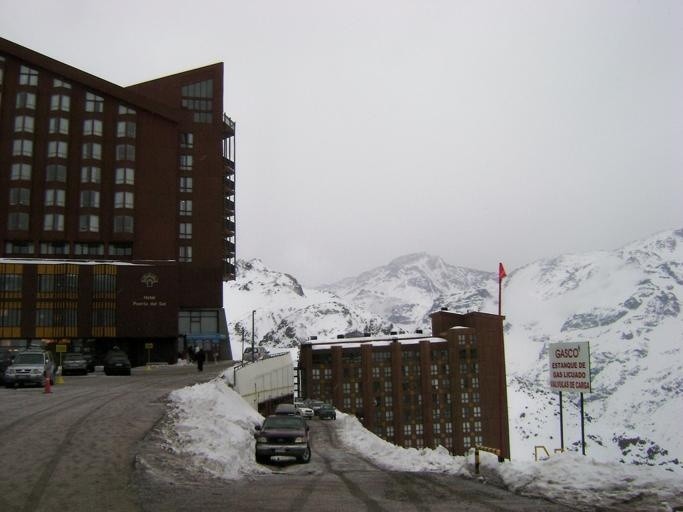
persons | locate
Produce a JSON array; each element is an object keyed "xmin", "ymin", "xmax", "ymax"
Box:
[{"xmin": 194, "ymin": 348, "xmax": 205, "ymax": 372}]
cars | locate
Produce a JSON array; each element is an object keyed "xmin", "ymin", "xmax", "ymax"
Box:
[
  {"xmin": 105, "ymin": 351, "xmax": 131, "ymax": 376},
  {"xmin": 62, "ymin": 353, "xmax": 94, "ymax": 374},
  {"xmin": 242, "ymin": 347, "xmax": 267, "ymax": 361},
  {"xmin": 275, "ymin": 399, "xmax": 336, "ymax": 420}
]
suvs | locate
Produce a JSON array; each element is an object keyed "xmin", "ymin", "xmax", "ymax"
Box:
[
  {"xmin": 254, "ymin": 415, "xmax": 311, "ymax": 464},
  {"xmin": 5, "ymin": 350, "xmax": 56, "ymax": 387}
]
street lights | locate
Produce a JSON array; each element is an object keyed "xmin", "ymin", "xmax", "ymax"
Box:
[{"xmin": 252, "ymin": 310, "xmax": 256, "ymax": 361}]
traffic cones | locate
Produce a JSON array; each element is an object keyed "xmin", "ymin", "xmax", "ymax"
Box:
[{"xmin": 42, "ymin": 372, "xmax": 52, "ymax": 394}]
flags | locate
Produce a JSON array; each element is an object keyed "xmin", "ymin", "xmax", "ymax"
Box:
[{"xmin": 498, "ymin": 264, "xmax": 506, "ymax": 279}]
